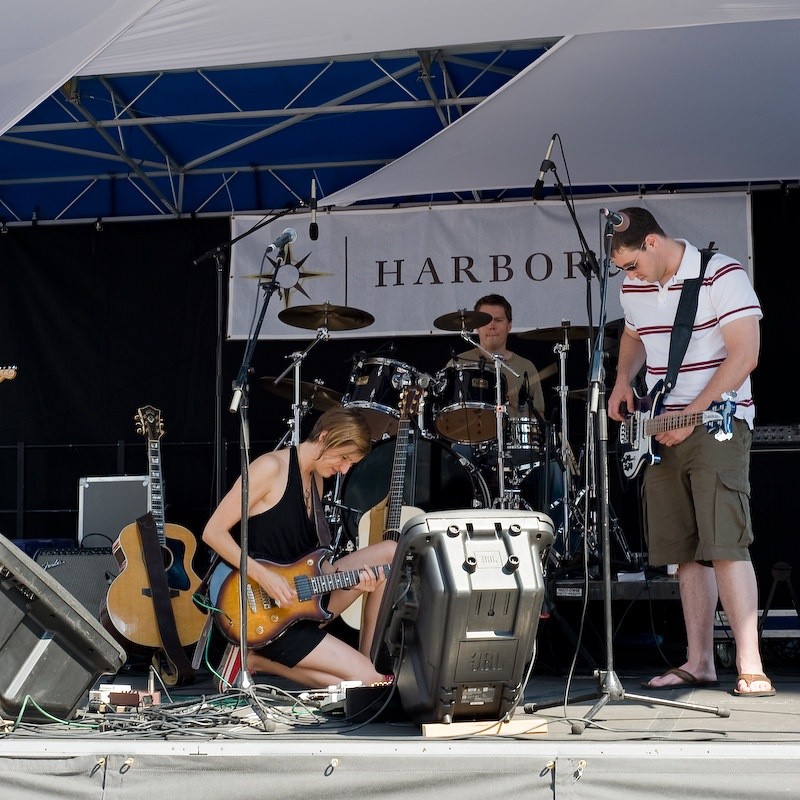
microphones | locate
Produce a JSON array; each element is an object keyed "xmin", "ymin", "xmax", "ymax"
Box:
[
  {"xmin": 532, "ymin": 134, "xmax": 558, "ymax": 201},
  {"xmin": 266, "ymin": 227, "xmax": 298, "ymax": 252},
  {"xmin": 309, "ymin": 173, "xmax": 319, "ymax": 241},
  {"xmin": 599, "ymin": 208, "xmax": 631, "ymax": 232}
]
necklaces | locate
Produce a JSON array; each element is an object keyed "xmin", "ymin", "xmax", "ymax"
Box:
[{"xmin": 296, "ymin": 444, "xmax": 313, "ymax": 513}]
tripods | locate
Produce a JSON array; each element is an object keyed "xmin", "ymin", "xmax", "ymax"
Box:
[
  {"xmin": 523, "ymin": 384, "xmax": 731, "ymax": 736},
  {"xmin": 146, "ymin": 396, "xmax": 325, "ymax": 734}
]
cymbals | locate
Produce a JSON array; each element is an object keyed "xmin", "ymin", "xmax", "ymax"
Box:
[
  {"xmin": 520, "ymin": 325, "xmax": 606, "ymax": 341},
  {"xmin": 433, "ymin": 309, "xmax": 493, "ymax": 332},
  {"xmin": 567, "ymin": 387, "xmax": 614, "ymax": 399},
  {"xmin": 278, "ymin": 303, "xmax": 375, "ymax": 331},
  {"xmin": 258, "ymin": 376, "xmax": 345, "ymax": 411}
]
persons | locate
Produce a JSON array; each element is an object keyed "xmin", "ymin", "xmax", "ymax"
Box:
[
  {"xmin": 603, "ymin": 206, "xmax": 777, "ymax": 698},
  {"xmin": 202, "ymin": 407, "xmax": 398, "ymax": 697},
  {"xmin": 445, "ymin": 294, "xmax": 576, "ymax": 566}
]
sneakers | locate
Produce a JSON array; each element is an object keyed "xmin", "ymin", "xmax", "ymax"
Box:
[{"xmin": 213, "ymin": 642, "xmax": 241, "ymax": 694}]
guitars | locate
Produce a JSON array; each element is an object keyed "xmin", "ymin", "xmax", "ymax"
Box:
[
  {"xmin": 617, "ymin": 380, "xmax": 737, "ymax": 480},
  {"xmin": 98, "ymin": 405, "xmax": 210, "ymax": 654},
  {"xmin": 216, "ymin": 548, "xmax": 394, "ymax": 649},
  {"xmin": 338, "ymin": 384, "xmax": 428, "ymax": 634}
]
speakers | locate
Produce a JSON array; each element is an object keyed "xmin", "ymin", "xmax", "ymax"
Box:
[
  {"xmin": 0, "ymin": 534, "xmax": 128, "ymax": 722},
  {"xmin": 32, "ymin": 547, "xmax": 153, "ymax": 678},
  {"xmin": 149, "ymin": 549, "xmax": 151, "ymax": 551},
  {"xmin": 715, "ymin": 425, "xmax": 800, "ymax": 610},
  {"xmin": 368, "ymin": 508, "xmax": 556, "ymax": 725}
]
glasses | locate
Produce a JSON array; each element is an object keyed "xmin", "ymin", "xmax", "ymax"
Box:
[{"xmin": 616, "ymin": 241, "xmax": 651, "ymax": 272}]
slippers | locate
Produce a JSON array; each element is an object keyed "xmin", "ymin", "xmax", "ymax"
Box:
[
  {"xmin": 640, "ymin": 668, "xmax": 720, "ymax": 690},
  {"xmin": 733, "ymin": 675, "xmax": 776, "ymax": 697}
]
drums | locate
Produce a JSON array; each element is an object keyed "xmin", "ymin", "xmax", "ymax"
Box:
[
  {"xmin": 434, "ymin": 362, "xmax": 509, "ymax": 447},
  {"xmin": 474, "ymin": 416, "xmax": 558, "ymax": 470},
  {"xmin": 344, "ymin": 357, "xmax": 436, "ymax": 441},
  {"xmin": 335, "ymin": 434, "xmax": 491, "ymax": 548}
]
